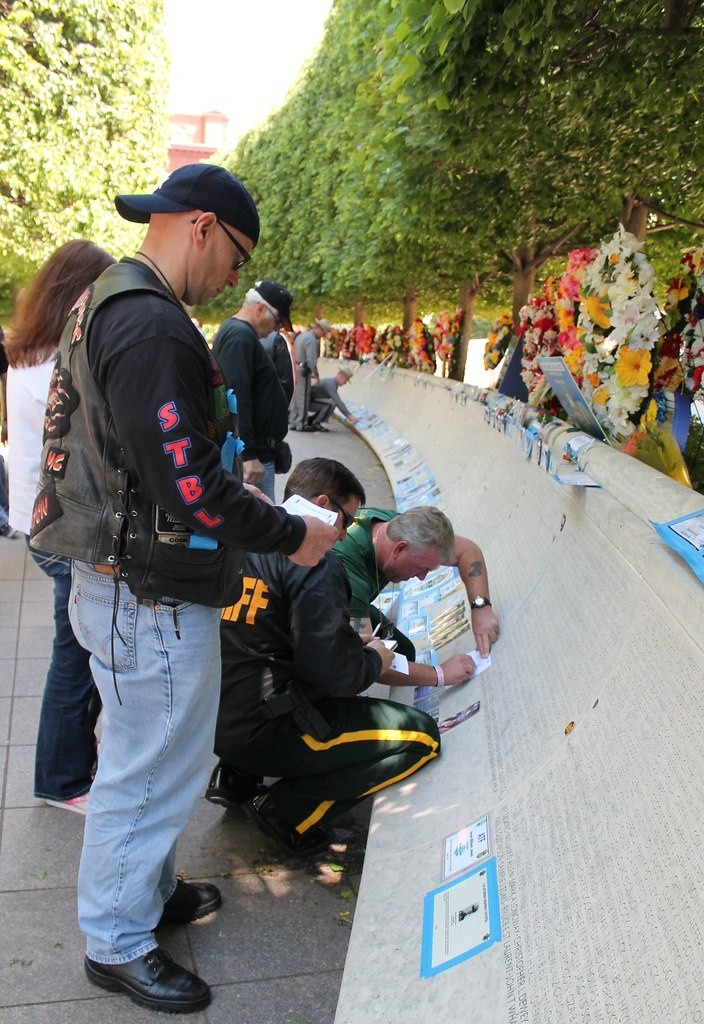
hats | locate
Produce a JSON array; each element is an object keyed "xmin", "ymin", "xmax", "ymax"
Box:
[
  {"xmin": 315, "ymin": 318, "xmax": 330, "ymax": 338},
  {"xmin": 338, "ymin": 366, "xmax": 353, "ymax": 384},
  {"xmin": 253, "ymin": 279, "xmax": 294, "ymax": 332},
  {"xmin": 115, "ymin": 164, "xmax": 261, "ymax": 249}
]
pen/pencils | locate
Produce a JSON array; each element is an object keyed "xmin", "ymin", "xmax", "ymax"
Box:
[{"xmin": 372, "ymin": 622, "xmax": 381, "ymax": 637}]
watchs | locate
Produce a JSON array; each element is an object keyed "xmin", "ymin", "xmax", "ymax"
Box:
[{"xmin": 471, "ymin": 595, "xmax": 492, "ymax": 609}]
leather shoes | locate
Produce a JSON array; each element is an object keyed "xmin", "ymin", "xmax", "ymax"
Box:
[
  {"xmin": 165, "ymin": 879, "xmax": 221, "ymax": 924},
  {"xmin": 205, "ymin": 768, "xmax": 269, "ymax": 809},
  {"xmin": 242, "ymin": 784, "xmax": 327, "ymax": 856},
  {"xmin": 85, "ymin": 948, "xmax": 211, "ymax": 1013}
]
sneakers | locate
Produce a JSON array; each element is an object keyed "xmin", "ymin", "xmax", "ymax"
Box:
[{"xmin": 45, "ymin": 793, "xmax": 88, "ymax": 816}]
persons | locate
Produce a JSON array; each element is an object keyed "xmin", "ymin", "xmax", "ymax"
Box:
[
  {"xmin": 308, "ymin": 366, "xmax": 359, "ymax": 432},
  {"xmin": 0, "ymin": 326, "xmax": 18, "ymax": 539},
  {"xmin": 289, "ymin": 318, "xmax": 330, "ymax": 432},
  {"xmin": 191, "ymin": 318, "xmax": 205, "ymax": 338},
  {"xmin": 2, "ymin": 239, "xmax": 116, "ymax": 815},
  {"xmin": 259, "ymin": 331, "xmax": 294, "ymax": 406},
  {"xmin": 331, "ymin": 506, "xmax": 499, "ymax": 686},
  {"xmin": 31, "ymin": 163, "xmax": 338, "ymax": 1014},
  {"xmin": 210, "ymin": 281, "xmax": 293, "ymax": 504},
  {"xmin": 207, "ymin": 457, "xmax": 441, "ymax": 855}
]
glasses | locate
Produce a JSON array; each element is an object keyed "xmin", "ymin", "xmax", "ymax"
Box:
[
  {"xmin": 191, "ymin": 215, "xmax": 252, "ymax": 270},
  {"xmin": 314, "ymin": 492, "xmax": 356, "ymax": 529},
  {"xmin": 257, "ymin": 302, "xmax": 284, "ymax": 329}
]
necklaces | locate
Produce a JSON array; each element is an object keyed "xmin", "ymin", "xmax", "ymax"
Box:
[{"xmin": 136, "ymin": 251, "xmax": 176, "ymax": 297}]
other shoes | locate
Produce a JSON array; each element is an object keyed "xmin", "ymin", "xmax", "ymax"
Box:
[
  {"xmin": 313, "ymin": 423, "xmax": 329, "ymax": 432},
  {"xmin": 304, "ymin": 426, "xmax": 315, "ymax": 431}
]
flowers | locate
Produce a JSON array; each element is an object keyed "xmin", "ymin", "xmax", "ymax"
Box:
[{"xmin": 322, "ymin": 222, "xmax": 704, "ymax": 440}]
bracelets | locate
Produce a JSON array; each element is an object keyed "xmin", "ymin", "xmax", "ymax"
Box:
[{"xmin": 433, "ymin": 666, "xmax": 444, "ymax": 687}]
